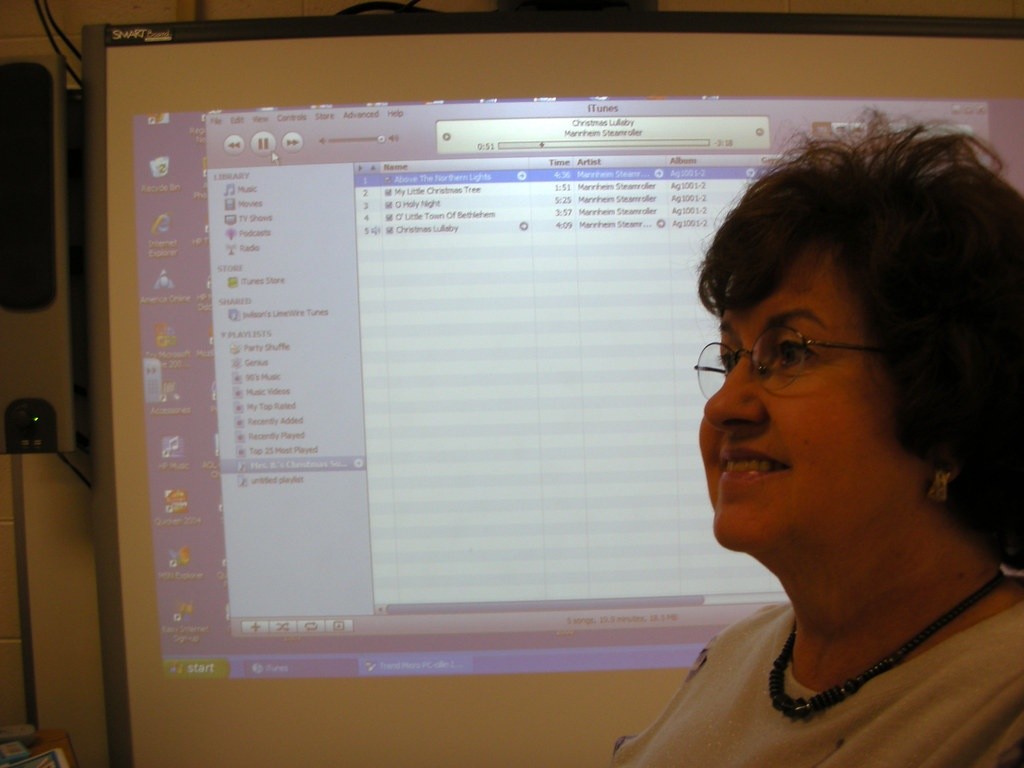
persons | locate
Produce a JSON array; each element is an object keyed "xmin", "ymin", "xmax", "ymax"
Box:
[{"xmin": 611, "ymin": 107, "xmax": 1023, "ymax": 768}]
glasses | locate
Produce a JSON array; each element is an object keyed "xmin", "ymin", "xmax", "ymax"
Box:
[{"xmin": 694, "ymin": 326, "xmax": 883, "ymax": 398}]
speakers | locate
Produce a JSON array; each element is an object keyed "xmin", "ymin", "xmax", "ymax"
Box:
[{"xmin": 0, "ymin": 61, "xmax": 54, "ymax": 312}]
